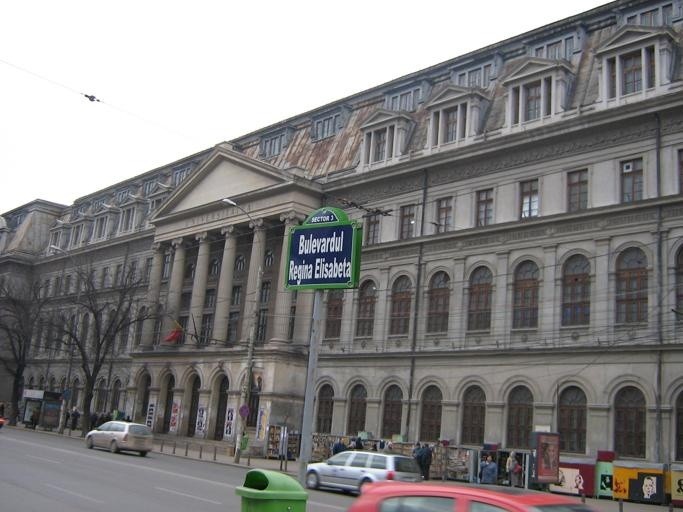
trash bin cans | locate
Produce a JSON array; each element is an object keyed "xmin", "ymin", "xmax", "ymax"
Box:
[
  {"xmin": 236, "ymin": 468, "xmax": 306, "ymax": 511},
  {"xmin": 594, "ymin": 461, "xmax": 614, "ymax": 500},
  {"xmin": 241, "ymin": 435, "xmax": 248, "ymax": 449}
]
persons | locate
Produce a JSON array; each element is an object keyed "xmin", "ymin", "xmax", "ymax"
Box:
[
  {"xmin": 643, "ymin": 477, "xmax": 654, "ymax": 498},
  {"xmin": 62, "ymin": 408, "xmax": 132, "ymax": 431},
  {"xmin": 677, "ymin": 479, "xmax": 683, "ymax": 493},
  {"xmin": 332, "ymin": 435, "xmax": 393, "ymax": 456},
  {"xmin": 601, "ymin": 475, "xmax": 612, "ymax": 491},
  {"xmin": 613, "ymin": 477, "xmax": 626, "ymax": 497},
  {"xmin": 478, "ymin": 451, "xmax": 522, "ymax": 487},
  {"xmin": 31, "ymin": 404, "xmax": 40, "ymax": 429},
  {"xmin": 412, "ymin": 442, "xmax": 434, "ymax": 481}
]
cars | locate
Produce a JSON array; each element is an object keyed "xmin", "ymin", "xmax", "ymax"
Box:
[
  {"xmin": 306, "ymin": 449, "xmax": 598, "ymax": 512},
  {"xmin": 84, "ymin": 421, "xmax": 154, "ymax": 456}
]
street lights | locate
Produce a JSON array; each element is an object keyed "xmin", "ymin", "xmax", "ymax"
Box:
[
  {"xmin": 223, "ymin": 197, "xmax": 263, "ymax": 463},
  {"xmin": 49, "ymin": 245, "xmax": 81, "ymax": 435}
]
